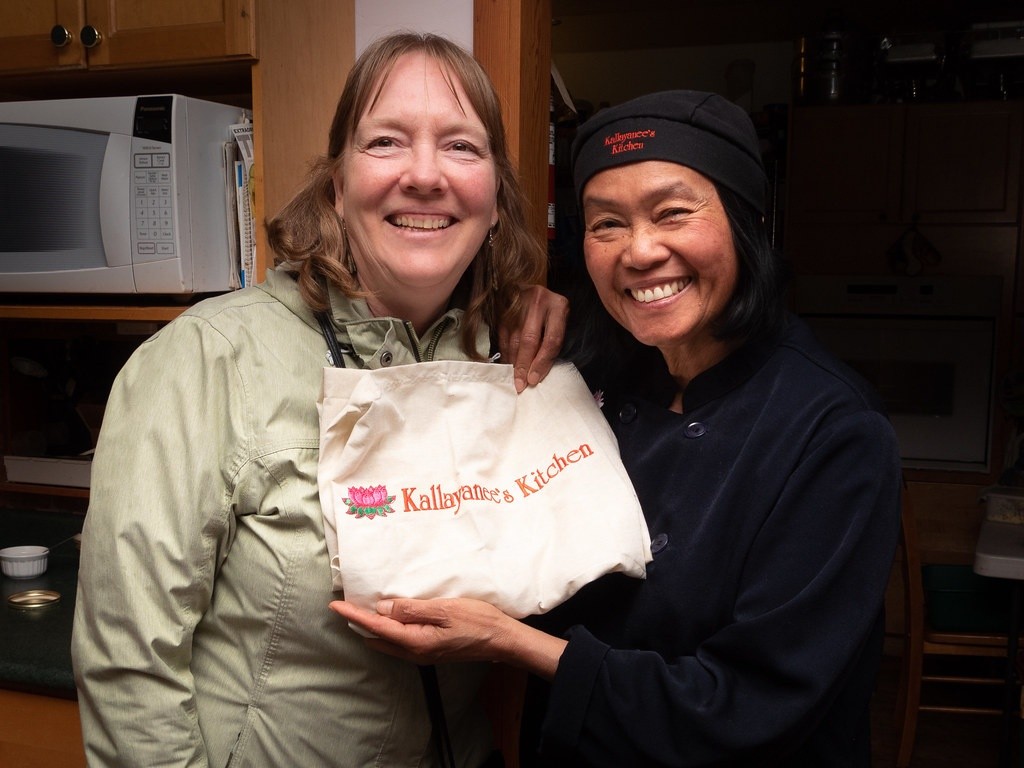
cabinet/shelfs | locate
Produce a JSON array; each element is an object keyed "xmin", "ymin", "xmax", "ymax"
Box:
[
  {"xmin": 0, "ymin": 2, "xmax": 357, "ymax": 495},
  {"xmin": 788, "ymin": 99, "xmax": 1024, "ymax": 657}
]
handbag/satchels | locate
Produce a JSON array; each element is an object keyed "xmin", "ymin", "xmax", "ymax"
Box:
[{"xmin": 322, "ymin": 357, "xmax": 652, "ymax": 636}]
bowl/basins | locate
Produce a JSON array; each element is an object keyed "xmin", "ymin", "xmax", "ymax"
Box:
[
  {"xmin": 7, "ymin": 590, "xmax": 63, "ymax": 619},
  {"xmin": 0, "ymin": 546, "xmax": 51, "ymax": 580}
]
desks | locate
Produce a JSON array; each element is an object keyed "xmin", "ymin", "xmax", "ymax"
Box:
[
  {"xmin": 972, "ymin": 493, "xmax": 1024, "ymax": 768},
  {"xmin": 0, "ymin": 508, "xmax": 90, "ymax": 768}
]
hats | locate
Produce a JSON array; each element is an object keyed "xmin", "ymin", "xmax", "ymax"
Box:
[{"xmin": 571, "ymin": 89, "xmax": 772, "ymax": 226}]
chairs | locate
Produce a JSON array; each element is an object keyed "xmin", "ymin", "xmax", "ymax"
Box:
[{"xmin": 893, "ymin": 475, "xmax": 1024, "ymax": 768}]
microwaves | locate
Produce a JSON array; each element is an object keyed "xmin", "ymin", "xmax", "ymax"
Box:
[{"xmin": 0, "ymin": 94, "xmax": 252, "ymax": 295}]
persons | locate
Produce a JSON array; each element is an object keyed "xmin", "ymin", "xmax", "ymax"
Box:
[
  {"xmin": 328, "ymin": 92, "xmax": 905, "ymax": 768},
  {"xmin": 71, "ymin": 33, "xmax": 549, "ymax": 768}
]
